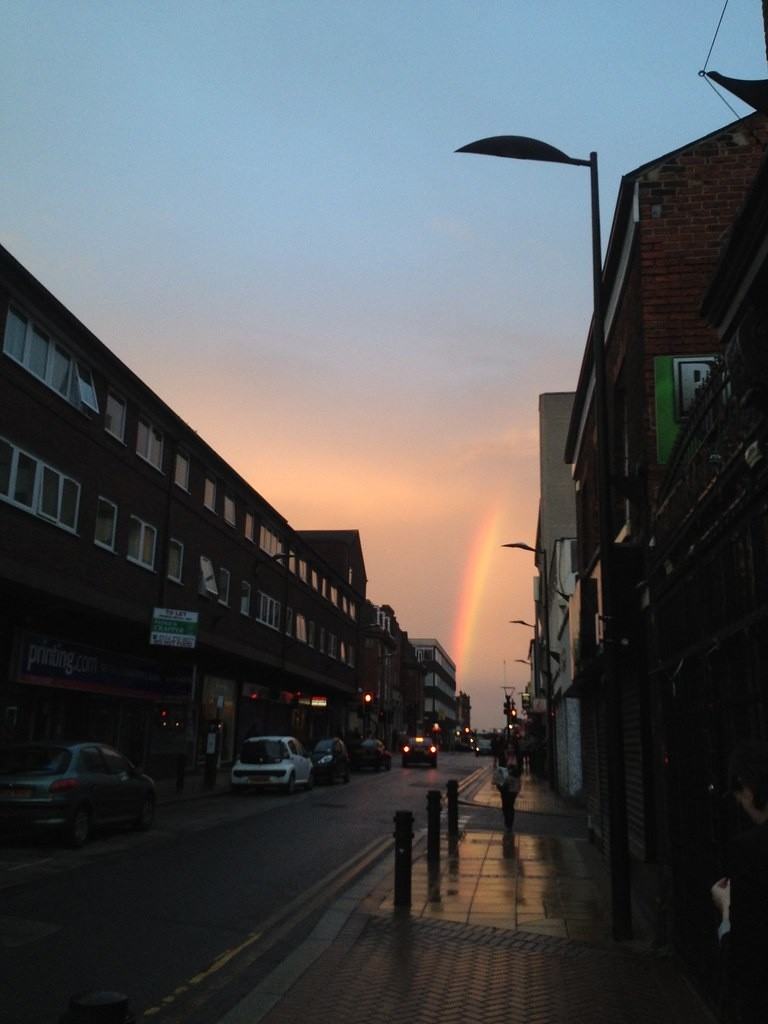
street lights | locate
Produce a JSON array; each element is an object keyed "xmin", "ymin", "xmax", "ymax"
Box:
[
  {"xmin": 501, "ymin": 543, "xmax": 550, "ymax": 780},
  {"xmin": 452, "ymin": 132, "xmax": 638, "ymax": 941}
]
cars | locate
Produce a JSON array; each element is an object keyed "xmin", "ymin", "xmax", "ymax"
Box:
[
  {"xmin": 402, "ymin": 737, "xmax": 439, "ymax": 769},
  {"xmin": 1, "ymin": 731, "xmax": 158, "ymax": 848},
  {"xmin": 475, "ymin": 740, "xmax": 494, "ymax": 757},
  {"xmin": 231, "ymin": 735, "xmax": 316, "ymax": 795},
  {"xmin": 349, "ymin": 737, "xmax": 392, "ymax": 773},
  {"xmin": 306, "ymin": 735, "xmax": 352, "ymax": 786}
]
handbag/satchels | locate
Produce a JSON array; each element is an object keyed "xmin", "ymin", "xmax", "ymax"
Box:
[{"xmin": 492, "ymin": 756, "xmax": 507, "ymax": 787}]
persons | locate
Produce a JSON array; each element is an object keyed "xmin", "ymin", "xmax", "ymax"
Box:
[
  {"xmin": 709, "ymin": 742, "xmax": 767, "ymax": 1024},
  {"xmin": 491, "ymin": 736, "xmax": 524, "ymax": 837}
]
